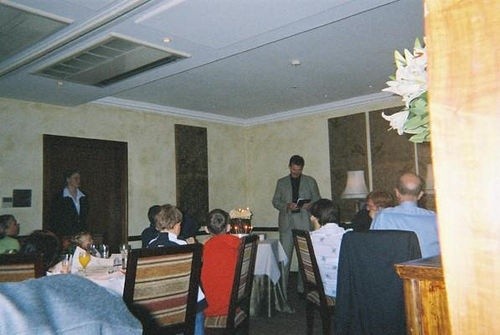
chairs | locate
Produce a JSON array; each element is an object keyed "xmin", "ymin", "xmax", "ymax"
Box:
[
  {"xmin": 291, "ymin": 228, "xmax": 336, "ymax": 335},
  {"xmin": 121, "ymin": 242, "xmax": 202, "ymax": 335},
  {"xmin": 206, "ymin": 233, "xmax": 259, "ymax": 335},
  {"xmin": 333, "ymin": 229, "xmax": 422, "ymax": 335},
  {"xmin": 0, "ymin": 251, "xmax": 45, "ymax": 284}
]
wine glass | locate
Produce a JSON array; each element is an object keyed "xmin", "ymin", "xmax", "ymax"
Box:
[{"xmin": 79, "ymin": 251, "xmax": 91, "ymax": 273}]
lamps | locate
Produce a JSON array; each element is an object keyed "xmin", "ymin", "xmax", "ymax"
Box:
[{"xmin": 342, "ymin": 170, "xmax": 370, "ymax": 213}]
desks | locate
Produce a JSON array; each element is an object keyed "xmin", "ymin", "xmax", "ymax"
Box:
[{"xmin": 392, "ymin": 253, "xmax": 452, "ymax": 335}]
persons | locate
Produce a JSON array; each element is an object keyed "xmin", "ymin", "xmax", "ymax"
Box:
[
  {"xmin": 23, "ymin": 231, "xmax": 101, "ymax": 277},
  {"xmin": 142, "ymin": 206, "xmax": 159, "ymax": 249},
  {"xmin": 369, "ymin": 172, "xmax": 440, "ymax": 259},
  {"xmin": 309, "ymin": 199, "xmax": 346, "ymax": 299},
  {"xmin": 49, "ymin": 168, "xmax": 90, "ymax": 234},
  {"xmin": 200, "ymin": 209, "xmax": 243, "ymax": 317},
  {"xmin": 272, "ymin": 155, "xmax": 320, "ymax": 301},
  {"xmin": 146, "ymin": 204, "xmax": 204, "ymax": 335},
  {"xmin": 0, "ymin": 214, "xmax": 21, "ymax": 255},
  {"xmin": 353, "ymin": 189, "xmax": 397, "ymax": 231}
]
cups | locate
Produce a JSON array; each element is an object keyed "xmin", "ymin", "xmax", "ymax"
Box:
[
  {"xmin": 62, "ymin": 254, "xmax": 72, "ymax": 273},
  {"xmin": 88, "ymin": 245, "xmax": 110, "ymax": 259},
  {"xmin": 120, "ymin": 243, "xmax": 128, "ymax": 254}
]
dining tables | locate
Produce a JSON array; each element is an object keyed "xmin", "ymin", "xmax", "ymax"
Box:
[
  {"xmin": 255, "ymin": 237, "xmax": 289, "ymax": 318},
  {"xmin": 47, "ymin": 253, "xmax": 127, "ymax": 296}
]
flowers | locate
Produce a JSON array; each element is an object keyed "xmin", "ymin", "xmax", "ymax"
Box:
[{"xmin": 380, "ymin": 34, "xmax": 431, "ymax": 144}]
